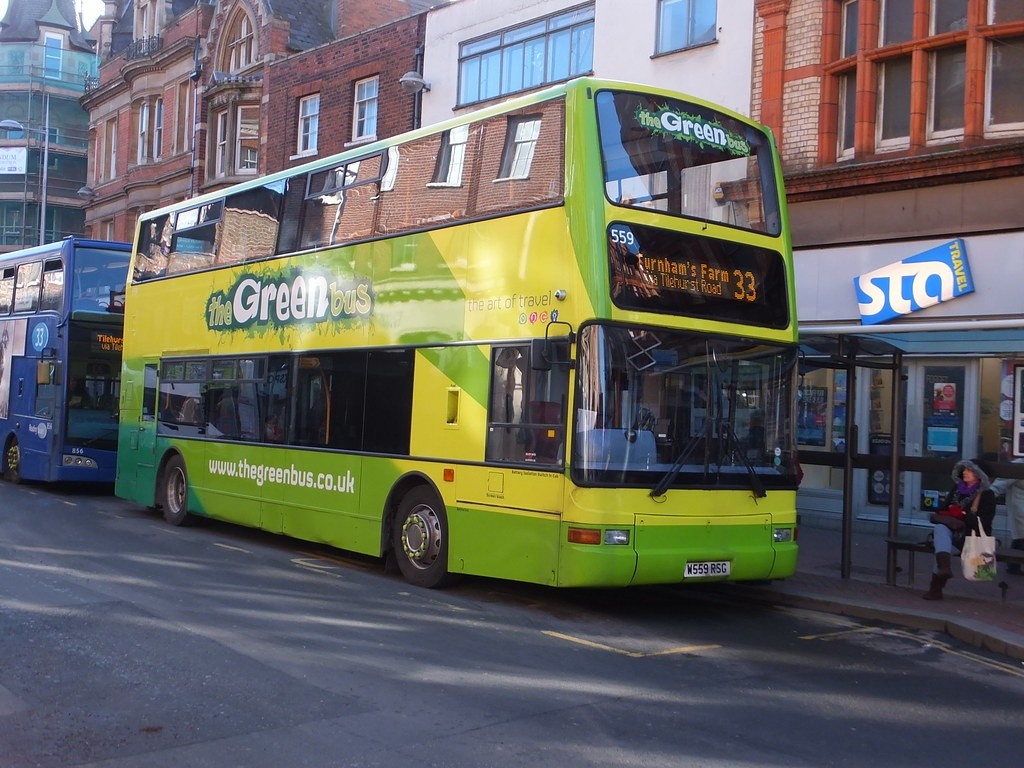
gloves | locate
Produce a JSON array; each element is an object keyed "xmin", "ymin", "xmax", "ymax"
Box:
[
  {"xmin": 939, "ymin": 510, "xmax": 950, "ymax": 515},
  {"xmin": 949, "ymin": 505, "xmax": 963, "ymax": 519}
]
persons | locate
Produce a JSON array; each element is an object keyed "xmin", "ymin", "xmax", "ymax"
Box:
[
  {"xmin": 718, "ymin": 423, "xmax": 773, "ymax": 469},
  {"xmin": 66, "ymin": 374, "xmax": 92, "ymax": 409},
  {"xmin": 921, "ymin": 457, "xmax": 995, "ymax": 600}
]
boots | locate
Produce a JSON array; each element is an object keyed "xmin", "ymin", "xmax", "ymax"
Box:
[
  {"xmin": 922, "ymin": 572, "xmax": 947, "ymax": 600},
  {"xmin": 935, "ymin": 552, "xmax": 954, "ymax": 579}
]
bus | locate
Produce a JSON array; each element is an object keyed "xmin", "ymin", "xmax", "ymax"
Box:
[
  {"xmin": 0, "ymin": 234, "xmax": 134, "ymax": 485},
  {"xmin": 112, "ymin": 74, "xmax": 806, "ymax": 598}
]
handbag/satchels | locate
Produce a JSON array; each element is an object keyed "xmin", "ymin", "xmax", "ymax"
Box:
[
  {"xmin": 960, "ymin": 516, "xmax": 997, "ymax": 581},
  {"xmin": 930, "ymin": 514, "xmax": 965, "ymax": 530}
]
